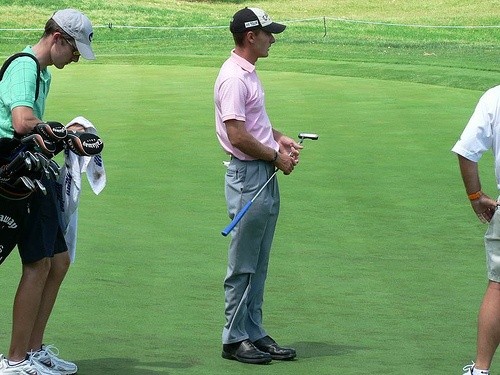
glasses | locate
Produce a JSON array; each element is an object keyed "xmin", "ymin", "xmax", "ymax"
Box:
[{"xmin": 55, "ymin": 29, "xmax": 82, "ymax": 56}]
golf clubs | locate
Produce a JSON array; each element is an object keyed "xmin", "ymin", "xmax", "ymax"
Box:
[
  {"xmin": 221, "ymin": 133, "xmax": 318, "ymax": 236},
  {"xmin": 11, "ymin": 150, "xmax": 61, "ymax": 196}
]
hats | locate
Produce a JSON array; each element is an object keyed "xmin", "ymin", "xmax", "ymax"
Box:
[
  {"xmin": 52, "ymin": 9, "xmax": 96, "ymax": 60},
  {"xmin": 230, "ymin": 7, "xmax": 287, "ymax": 34}
]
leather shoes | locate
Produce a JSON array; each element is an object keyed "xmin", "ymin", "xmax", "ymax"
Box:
[
  {"xmin": 253, "ymin": 335, "xmax": 297, "ymax": 360},
  {"xmin": 222, "ymin": 338, "xmax": 272, "ymax": 363}
]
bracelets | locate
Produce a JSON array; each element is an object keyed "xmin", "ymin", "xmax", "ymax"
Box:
[
  {"xmin": 272, "ymin": 152, "xmax": 277, "ymax": 161},
  {"xmin": 468, "ymin": 191, "xmax": 481, "ymax": 200}
]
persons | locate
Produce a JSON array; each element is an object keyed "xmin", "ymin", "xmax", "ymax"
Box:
[
  {"xmin": 213, "ymin": 6, "xmax": 304, "ymax": 363},
  {"xmin": 0, "ymin": 8, "xmax": 105, "ymax": 375},
  {"xmin": 450, "ymin": 83, "xmax": 500, "ymax": 375}
]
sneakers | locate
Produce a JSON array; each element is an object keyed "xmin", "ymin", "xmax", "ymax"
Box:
[
  {"xmin": 462, "ymin": 360, "xmax": 476, "ymax": 375},
  {"xmin": 27, "ymin": 343, "xmax": 78, "ymax": 375},
  {"xmin": 0, "ymin": 354, "xmax": 66, "ymax": 375}
]
